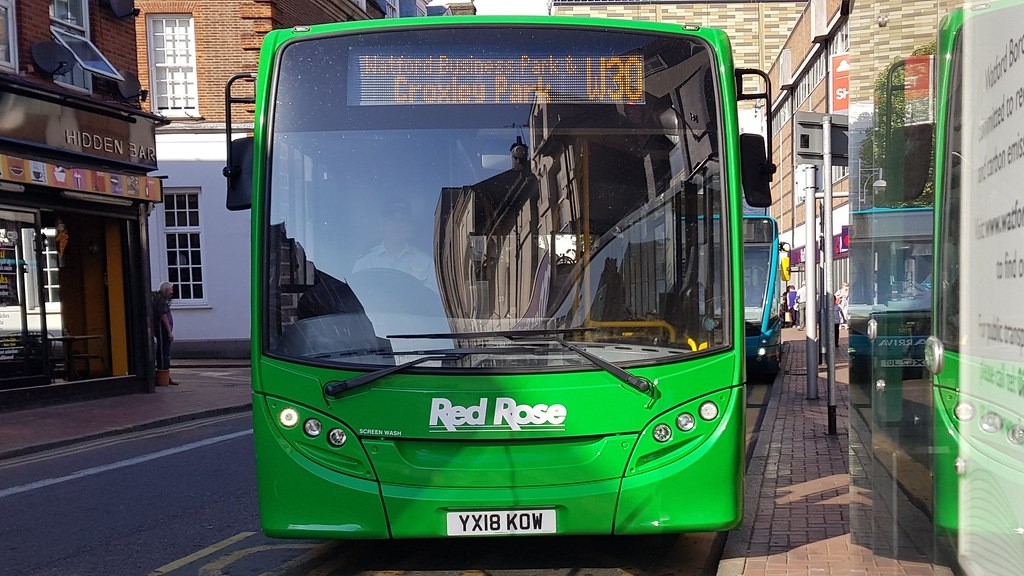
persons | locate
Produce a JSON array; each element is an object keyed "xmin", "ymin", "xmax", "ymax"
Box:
[
  {"xmin": 153, "ymin": 281, "xmax": 180, "ymax": 385},
  {"xmin": 794, "ymin": 280, "xmax": 806, "ymax": 331},
  {"xmin": 833, "ymin": 295, "xmax": 847, "ymax": 347},
  {"xmin": 834, "ymin": 283, "xmax": 848, "ymax": 330},
  {"xmin": 783, "ymin": 285, "xmax": 800, "ymax": 328},
  {"xmin": 352, "ymin": 200, "xmax": 440, "ymax": 294}
]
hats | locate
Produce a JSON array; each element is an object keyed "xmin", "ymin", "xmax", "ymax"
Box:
[{"xmin": 802, "ymin": 281, "xmax": 805, "ymax": 284}]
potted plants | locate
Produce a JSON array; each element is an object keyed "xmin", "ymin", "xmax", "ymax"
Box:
[{"xmin": 150, "ymin": 290, "xmax": 170, "ymax": 386}]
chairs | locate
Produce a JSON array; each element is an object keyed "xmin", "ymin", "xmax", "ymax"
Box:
[{"xmin": 29, "ymin": 332, "xmax": 109, "ymax": 381}]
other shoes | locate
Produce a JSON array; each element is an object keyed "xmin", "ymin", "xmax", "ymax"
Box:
[
  {"xmin": 836, "ymin": 344, "xmax": 839, "ymax": 347},
  {"xmin": 169, "ymin": 377, "xmax": 179, "ymax": 385}
]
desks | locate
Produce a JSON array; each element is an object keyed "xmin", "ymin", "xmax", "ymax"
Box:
[{"xmin": 47, "ymin": 334, "xmax": 102, "ymax": 381}]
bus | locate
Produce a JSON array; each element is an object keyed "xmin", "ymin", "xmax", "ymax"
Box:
[
  {"xmin": 851, "ymin": 208, "xmax": 934, "ymax": 382},
  {"xmin": 681, "ymin": 211, "xmax": 791, "ymax": 382},
  {"xmin": 879, "ymin": 2, "xmax": 1023, "ymax": 533},
  {"xmin": 222, "ymin": 15, "xmax": 776, "ymax": 541}
]
